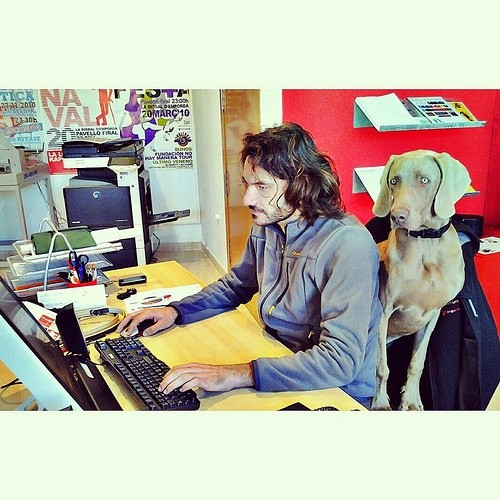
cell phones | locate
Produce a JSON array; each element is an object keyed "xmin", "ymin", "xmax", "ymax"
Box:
[{"xmin": 119, "ymin": 274, "xmax": 147, "ymax": 286}]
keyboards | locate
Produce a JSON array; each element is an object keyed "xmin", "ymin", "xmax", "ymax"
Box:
[{"xmin": 94, "ymin": 334, "xmax": 201, "ymax": 411}]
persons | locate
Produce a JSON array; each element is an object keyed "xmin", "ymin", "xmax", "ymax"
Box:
[{"xmin": 114, "ymin": 120, "xmax": 382, "ymax": 411}]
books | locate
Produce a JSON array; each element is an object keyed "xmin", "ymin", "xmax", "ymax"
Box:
[{"xmin": 355, "ymin": 93, "xmax": 467, "ymax": 130}]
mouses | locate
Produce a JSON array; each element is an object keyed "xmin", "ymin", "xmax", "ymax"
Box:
[{"xmin": 120, "ymin": 318, "xmax": 173, "ymax": 337}]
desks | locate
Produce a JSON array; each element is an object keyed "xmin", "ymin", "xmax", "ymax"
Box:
[
  {"xmin": 0, "ymin": 260, "xmax": 369, "ymax": 411},
  {"xmin": 474, "ymin": 228, "xmax": 500, "ymax": 340},
  {"xmin": 0, "ymin": 173, "xmax": 57, "ymax": 250}
]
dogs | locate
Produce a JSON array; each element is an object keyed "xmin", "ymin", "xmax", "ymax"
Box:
[{"xmin": 369, "ymin": 146, "xmax": 473, "ymax": 412}]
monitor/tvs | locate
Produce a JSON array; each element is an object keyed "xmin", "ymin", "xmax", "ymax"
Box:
[
  {"xmin": 62, "ymin": 186, "xmax": 131, "ymax": 234},
  {"xmin": 0, "ymin": 278, "xmax": 126, "ymax": 411}
]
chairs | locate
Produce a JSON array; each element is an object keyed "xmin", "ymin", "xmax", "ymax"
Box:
[{"xmin": 363, "ymin": 212, "xmax": 499, "ymax": 411}]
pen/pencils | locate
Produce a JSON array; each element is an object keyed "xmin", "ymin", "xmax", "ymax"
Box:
[
  {"xmin": 56, "ymin": 260, "xmax": 98, "ymax": 285},
  {"xmin": 86, "ymin": 334, "xmax": 105, "ymax": 342}
]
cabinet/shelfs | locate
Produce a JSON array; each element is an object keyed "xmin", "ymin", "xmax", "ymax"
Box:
[
  {"xmin": 351, "ymin": 101, "xmax": 487, "ymax": 206},
  {"xmin": 4, "ymin": 239, "xmax": 118, "ymax": 297}
]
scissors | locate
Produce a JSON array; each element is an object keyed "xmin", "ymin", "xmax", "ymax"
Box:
[{"xmin": 69, "ymin": 251, "xmax": 89, "ymax": 281}]
